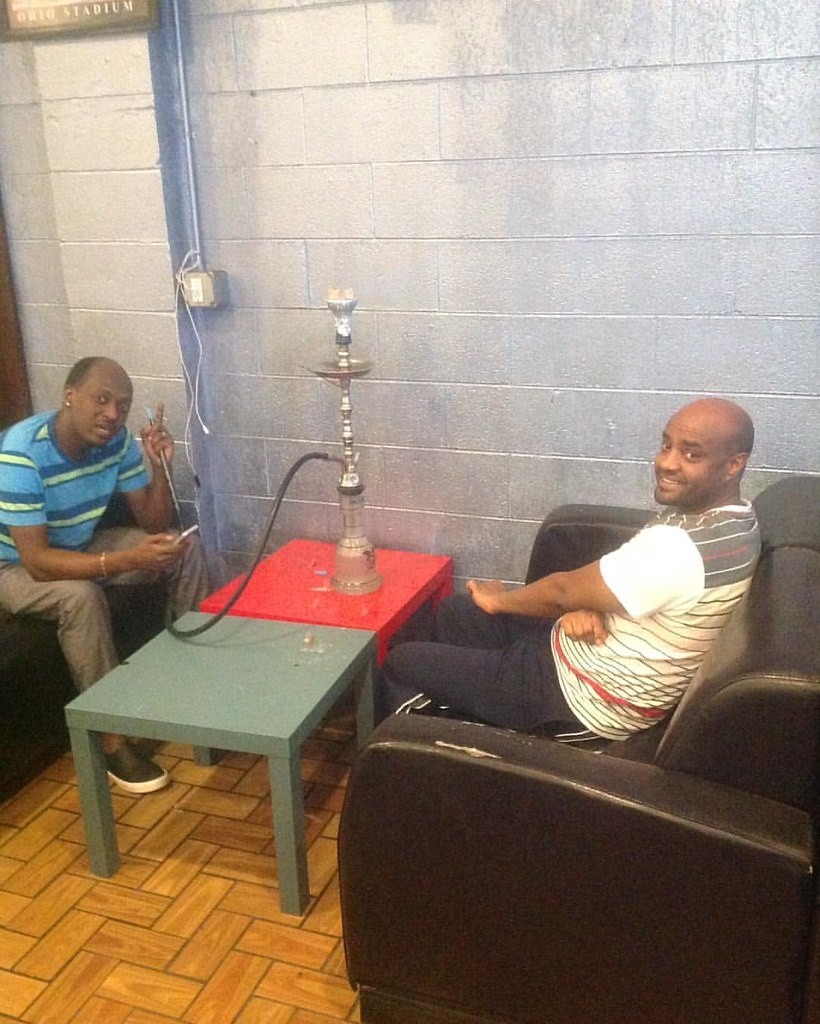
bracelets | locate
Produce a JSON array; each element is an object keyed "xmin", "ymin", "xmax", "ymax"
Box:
[{"xmin": 100, "ymin": 552, "xmax": 109, "ymax": 579}]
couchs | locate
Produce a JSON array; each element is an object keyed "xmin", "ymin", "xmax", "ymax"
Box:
[
  {"xmin": 337, "ymin": 476, "xmax": 820, "ymax": 1024},
  {"xmin": 0, "ymin": 494, "xmax": 168, "ymax": 801}
]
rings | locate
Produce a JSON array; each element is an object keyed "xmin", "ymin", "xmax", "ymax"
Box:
[{"xmin": 162, "ymin": 432, "xmax": 167, "ymax": 437}]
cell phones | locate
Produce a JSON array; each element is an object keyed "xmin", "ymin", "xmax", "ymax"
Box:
[{"xmin": 173, "ymin": 525, "xmax": 199, "ymax": 545}]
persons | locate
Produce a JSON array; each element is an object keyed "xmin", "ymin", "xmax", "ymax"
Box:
[
  {"xmin": 0, "ymin": 356, "xmax": 210, "ymax": 794},
  {"xmin": 379, "ymin": 398, "xmax": 762, "ymax": 744}
]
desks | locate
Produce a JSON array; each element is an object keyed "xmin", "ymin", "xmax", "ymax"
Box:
[
  {"xmin": 62, "ymin": 610, "xmax": 379, "ymax": 916},
  {"xmin": 201, "ymin": 537, "xmax": 454, "ymax": 665}
]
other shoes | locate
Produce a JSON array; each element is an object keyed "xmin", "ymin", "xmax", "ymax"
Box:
[{"xmin": 103, "ymin": 743, "xmax": 171, "ymax": 794}]
